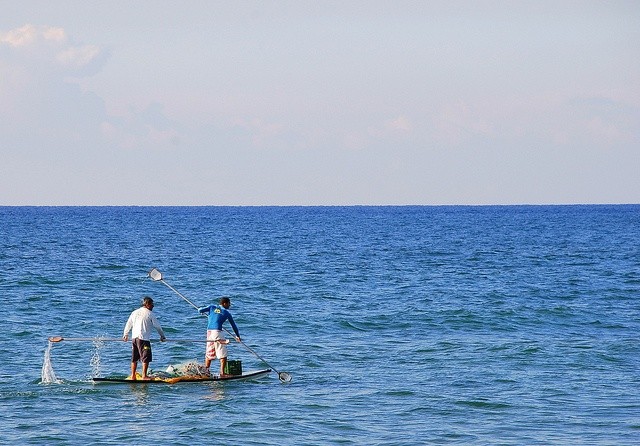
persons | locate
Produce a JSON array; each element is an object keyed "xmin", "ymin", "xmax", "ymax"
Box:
[
  {"xmin": 121, "ymin": 297, "xmax": 166, "ymax": 380},
  {"xmin": 198, "ymin": 297, "xmax": 241, "ymax": 379}
]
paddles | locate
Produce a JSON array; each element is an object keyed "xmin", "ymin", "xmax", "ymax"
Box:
[
  {"xmin": 48, "ymin": 336, "xmax": 230, "ymax": 344},
  {"xmin": 149, "ymin": 268, "xmax": 292, "ymax": 383}
]
